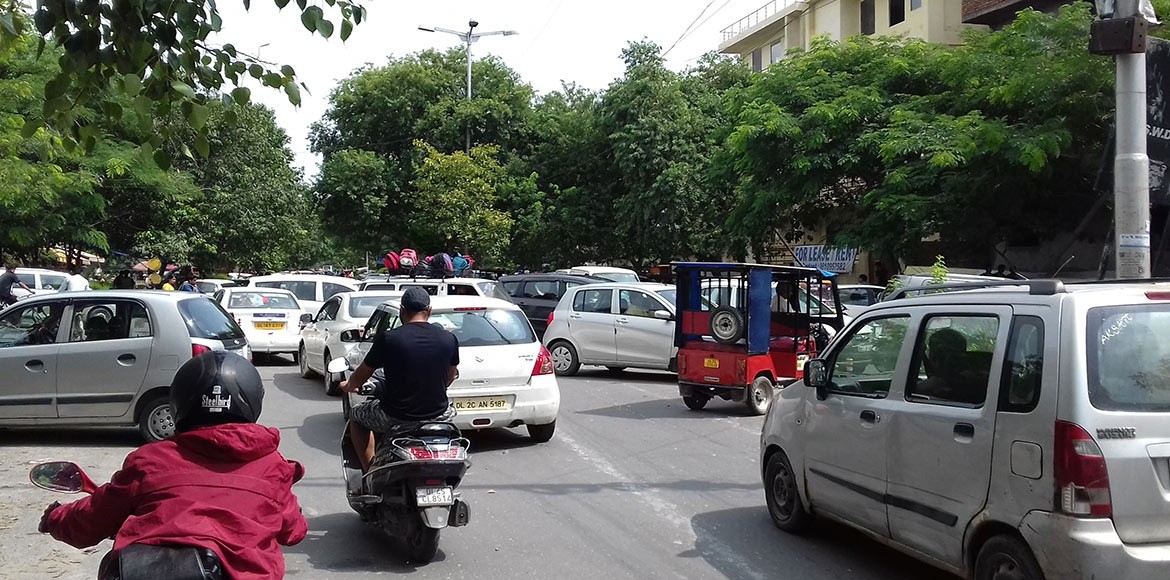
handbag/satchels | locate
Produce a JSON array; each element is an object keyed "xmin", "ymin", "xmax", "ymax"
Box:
[{"xmin": 383, "ymin": 248, "xmax": 476, "ymax": 278}]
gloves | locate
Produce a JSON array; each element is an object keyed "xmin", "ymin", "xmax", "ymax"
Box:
[{"xmin": 38, "ymin": 501, "xmax": 61, "ymax": 533}]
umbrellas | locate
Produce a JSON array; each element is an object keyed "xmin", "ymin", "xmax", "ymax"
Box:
[{"xmin": 133, "ymin": 260, "xmax": 150, "ymax": 276}]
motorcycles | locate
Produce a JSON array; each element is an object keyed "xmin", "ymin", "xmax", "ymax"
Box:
[{"xmin": 667, "ymin": 260, "xmax": 846, "ymax": 416}]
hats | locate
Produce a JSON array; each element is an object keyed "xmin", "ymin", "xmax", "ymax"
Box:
[{"xmin": 401, "ymin": 287, "xmax": 430, "ymax": 311}]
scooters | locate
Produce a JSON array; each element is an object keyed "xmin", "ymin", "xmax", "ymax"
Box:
[
  {"xmin": 28, "ymin": 460, "xmax": 305, "ymax": 580},
  {"xmin": 326, "ymin": 355, "xmax": 474, "ymax": 566}
]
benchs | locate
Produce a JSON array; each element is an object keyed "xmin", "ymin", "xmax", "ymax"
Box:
[{"xmin": 769, "ymin": 336, "xmax": 817, "ymax": 354}]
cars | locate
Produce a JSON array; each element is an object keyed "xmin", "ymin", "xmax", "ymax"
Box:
[
  {"xmin": 339, "ymin": 294, "xmax": 562, "ymax": 443},
  {"xmin": 542, "ymin": 281, "xmax": 747, "ymax": 384},
  {"xmin": 0, "ymin": 266, "xmax": 116, "ymax": 324},
  {"xmin": 0, "ymin": 288, "xmax": 253, "ymax": 444},
  {"xmin": 297, "ymin": 289, "xmax": 459, "ymax": 397},
  {"xmin": 211, "ymin": 286, "xmax": 310, "ymax": 365},
  {"xmin": 196, "ymin": 268, "xmax": 1003, "ymax": 375}
]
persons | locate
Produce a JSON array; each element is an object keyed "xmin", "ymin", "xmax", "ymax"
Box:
[
  {"xmin": 980, "ymin": 261, "xmax": 993, "ymax": 276},
  {"xmin": 340, "ymin": 287, "xmax": 460, "ymax": 494},
  {"xmin": 913, "ymin": 327, "xmax": 968, "ymax": 393},
  {"xmin": 37, "ymin": 349, "xmax": 311, "ymax": 580},
  {"xmin": 992, "ymin": 263, "xmax": 1007, "ymax": 278},
  {"xmin": 178, "ymin": 275, "xmax": 205, "ymax": 294},
  {"xmin": 158, "ymin": 272, "xmax": 176, "ymax": 291},
  {"xmin": 113, "ymin": 269, "xmax": 135, "ymax": 290},
  {"xmin": 72, "ymin": 264, "xmax": 83, "ymax": 274},
  {"xmin": 0, "ymin": 263, "xmax": 36, "ymax": 307},
  {"xmin": 770, "ymin": 282, "xmax": 806, "ymax": 313},
  {"xmin": 1005, "ymin": 265, "xmax": 1020, "ymax": 279}
]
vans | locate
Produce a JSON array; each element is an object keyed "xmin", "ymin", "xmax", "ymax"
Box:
[{"xmin": 759, "ymin": 279, "xmax": 1170, "ymax": 580}]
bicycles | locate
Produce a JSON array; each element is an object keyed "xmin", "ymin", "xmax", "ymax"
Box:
[{"xmin": 0, "ymin": 293, "xmax": 48, "ymax": 330}]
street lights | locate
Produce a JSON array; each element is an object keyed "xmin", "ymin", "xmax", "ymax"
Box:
[{"xmin": 417, "ymin": 18, "xmax": 521, "ymax": 157}]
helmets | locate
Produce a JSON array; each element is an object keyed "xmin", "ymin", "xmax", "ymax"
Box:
[{"xmin": 169, "ymin": 350, "xmax": 264, "ymax": 431}]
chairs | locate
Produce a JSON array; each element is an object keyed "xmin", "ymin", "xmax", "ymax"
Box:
[
  {"xmin": 86, "ymin": 316, "xmax": 109, "ymax": 341},
  {"xmin": 621, "ymin": 299, "xmax": 628, "ymax": 315},
  {"xmin": 106, "ymin": 317, "xmax": 125, "ymax": 339},
  {"xmin": 930, "ymin": 351, "xmax": 995, "ymax": 402}
]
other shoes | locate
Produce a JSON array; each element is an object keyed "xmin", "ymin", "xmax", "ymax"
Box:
[{"xmin": 348, "ymin": 486, "xmax": 367, "ymax": 496}]
